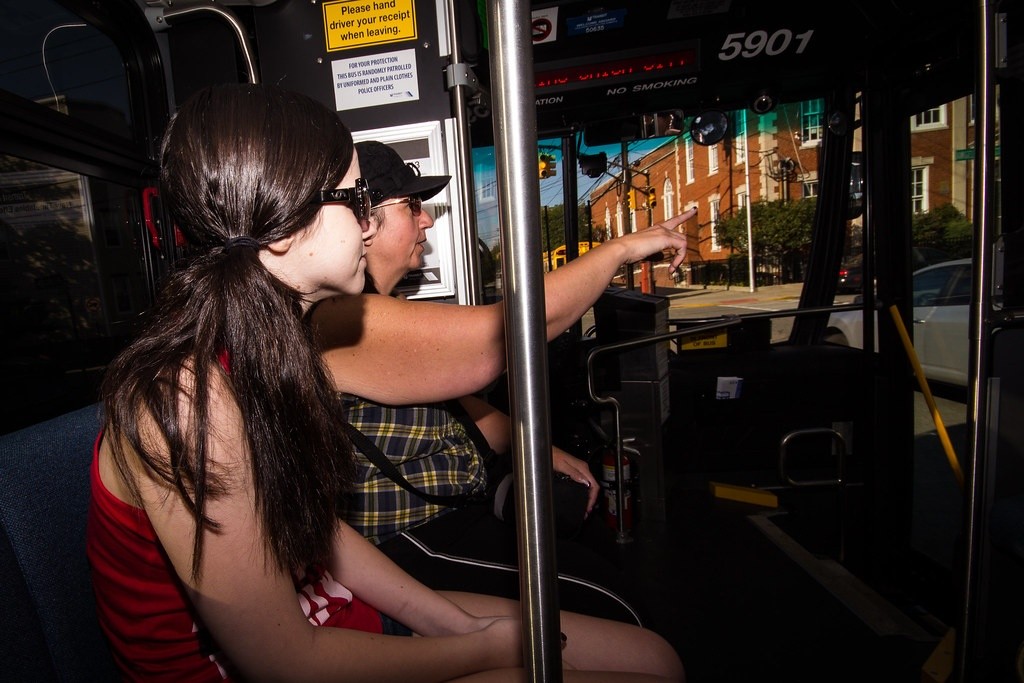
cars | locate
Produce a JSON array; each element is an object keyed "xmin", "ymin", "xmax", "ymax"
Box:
[{"xmin": 825, "ymin": 255, "xmax": 971, "ymax": 389}]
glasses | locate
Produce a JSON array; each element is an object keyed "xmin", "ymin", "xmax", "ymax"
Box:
[
  {"xmin": 312, "ymin": 174, "xmax": 372, "ymax": 232},
  {"xmin": 370, "ymin": 192, "xmax": 422, "ymax": 217}
]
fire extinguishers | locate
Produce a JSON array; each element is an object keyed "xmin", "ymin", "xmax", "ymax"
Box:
[{"xmin": 586, "ymin": 416, "xmax": 642, "ymax": 530}]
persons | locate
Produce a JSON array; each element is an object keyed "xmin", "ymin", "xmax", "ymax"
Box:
[
  {"xmin": 302, "ymin": 140, "xmax": 700, "ymax": 628},
  {"xmin": 85, "ymin": 84, "xmax": 686, "ymax": 683}
]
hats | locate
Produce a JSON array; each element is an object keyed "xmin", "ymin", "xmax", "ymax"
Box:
[{"xmin": 354, "ymin": 141, "xmax": 451, "ymax": 207}]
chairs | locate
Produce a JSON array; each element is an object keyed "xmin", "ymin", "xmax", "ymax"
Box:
[{"xmin": 1, "ymin": 392, "xmax": 117, "ymax": 683}]
